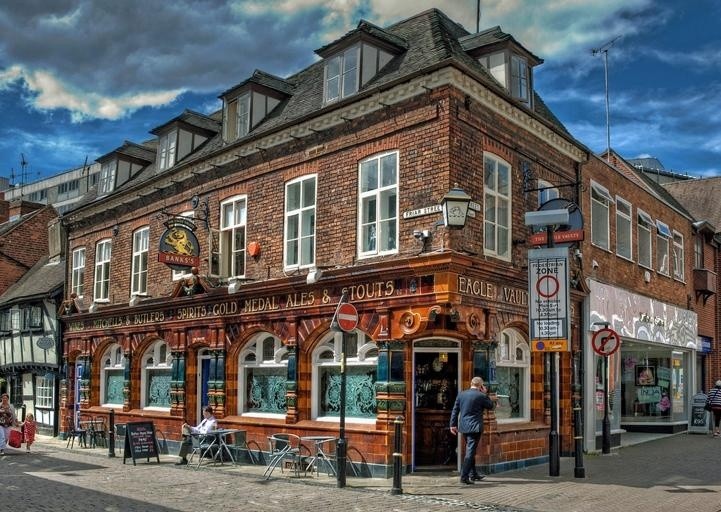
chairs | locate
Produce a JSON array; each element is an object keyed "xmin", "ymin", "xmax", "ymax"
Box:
[
  {"xmin": 263, "ymin": 433, "xmax": 311, "ymax": 480},
  {"xmin": 114, "ymin": 423, "xmax": 127, "ymax": 454},
  {"xmin": 187, "ymin": 427, "xmax": 256, "ymax": 470},
  {"xmin": 305, "ymin": 436, "xmax": 358, "ymax": 478},
  {"xmin": 66, "ymin": 413, "xmax": 108, "ymax": 450},
  {"xmin": 154, "ymin": 425, "xmax": 162, "ymax": 453}
]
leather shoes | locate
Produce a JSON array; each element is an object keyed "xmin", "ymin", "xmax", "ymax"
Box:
[
  {"xmin": 471, "ymin": 475, "xmax": 485, "ymax": 481},
  {"xmin": 460, "ymin": 479, "xmax": 474, "ymax": 484},
  {"xmin": 176, "ymin": 460, "xmax": 187, "ymax": 465}
]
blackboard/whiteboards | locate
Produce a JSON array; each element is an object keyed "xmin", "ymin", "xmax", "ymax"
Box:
[{"xmin": 127, "ymin": 421, "xmax": 157, "ymax": 458}]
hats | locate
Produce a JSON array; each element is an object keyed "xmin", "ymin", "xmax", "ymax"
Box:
[{"xmin": 715, "ymin": 380, "xmax": 721, "ymax": 387}]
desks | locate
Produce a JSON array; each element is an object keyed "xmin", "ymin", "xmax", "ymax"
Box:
[{"xmin": 299, "ymin": 435, "xmax": 336, "ymax": 477}]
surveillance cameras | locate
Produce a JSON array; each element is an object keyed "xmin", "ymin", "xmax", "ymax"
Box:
[{"xmin": 414, "ymin": 229, "xmax": 421, "ymax": 239}]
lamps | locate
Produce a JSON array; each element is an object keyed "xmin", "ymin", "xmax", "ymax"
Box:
[
  {"xmin": 228, "ymin": 278, "xmax": 255, "ymax": 294},
  {"xmin": 438, "ymin": 352, "xmax": 448, "ymax": 363},
  {"xmin": 307, "ymin": 266, "xmax": 337, "ymax": 284},
  {"xmin": 129, "ymin": 295, "xmax": 153, "ymax": 307},
  {"xmin": 89, "ymin": 302, "xmax": 110, "ymax": 313},
  {"xmin": 440, "ymin": 182, "xmax": 472, "ymax": 229}
]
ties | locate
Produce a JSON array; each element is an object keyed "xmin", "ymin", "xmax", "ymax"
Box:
[{"xmin": 198, "ymin": 420, "xmax": 208, "ymax": 429}]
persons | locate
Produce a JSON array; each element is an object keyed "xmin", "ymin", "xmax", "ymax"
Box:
[
  {"xmin": 0, "ymin": 393, "xmax": 14, "ymax": 456},
  {"xmin": 175, "ymin": 405, "xmax": 217, "ymax": 465},
  {"xmin": 450, "ymin": 377, "xmax": 493, "ymax": 484},
  {"xmin": 706, "ymin": 380, "xmax": 721, "ymax": 437},
  {"xmin": 17, "ymin": 413, "xmax": 38, "ymax": 453}
]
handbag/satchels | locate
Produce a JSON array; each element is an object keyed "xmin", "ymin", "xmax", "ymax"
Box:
[
  {"xmin": 704, "ymin": 403, "xmax": 712, "ymax": 412},
  {"xmin": 9, "ymin": 427, "xmax": 23, "ymax": 448}
]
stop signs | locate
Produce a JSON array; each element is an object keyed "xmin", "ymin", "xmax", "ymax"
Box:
[{"xmin": 336, "ymin": 303, "xmax": 359, "ymax": 332}]
